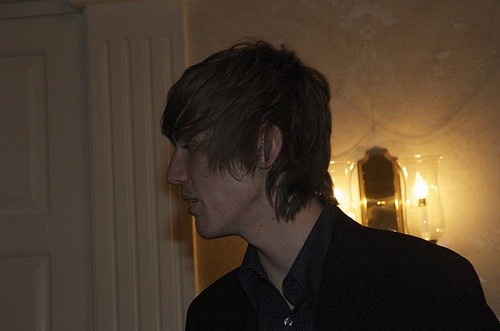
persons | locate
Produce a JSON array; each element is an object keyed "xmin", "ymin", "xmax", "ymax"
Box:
[{"xmin": 155, "ymin": 40, "xmax": 500, "ymax": 330}]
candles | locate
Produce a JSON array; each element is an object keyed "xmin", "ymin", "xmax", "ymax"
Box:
[{"xmin": 413, "ymin": 170, "xmax": 431, "ymax": 241}]
